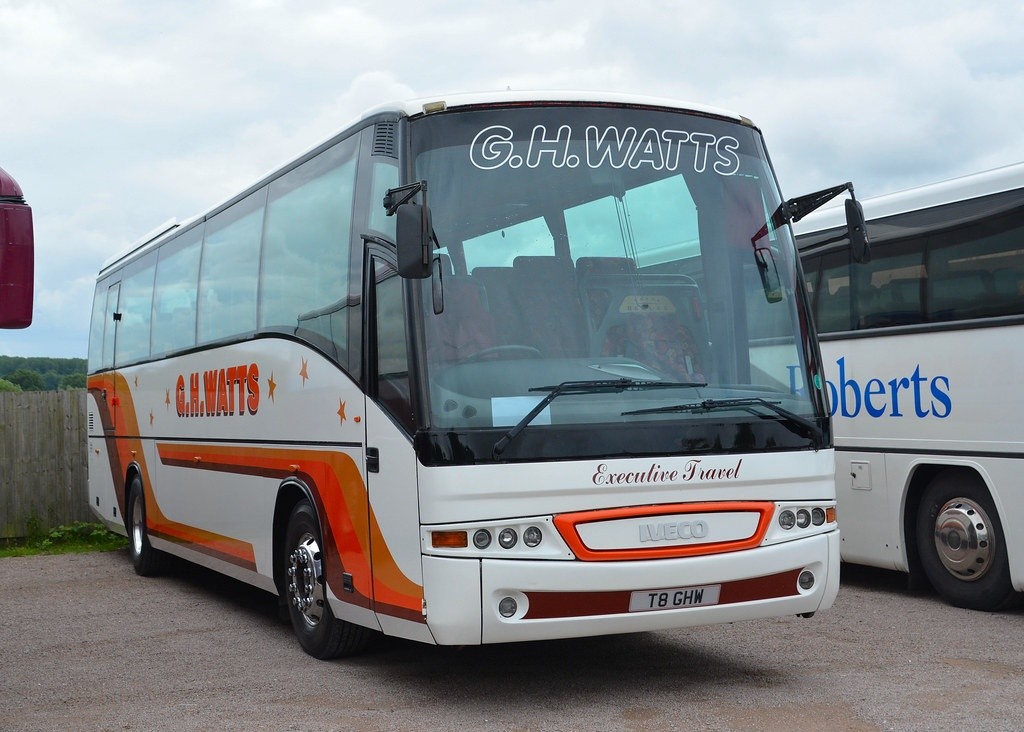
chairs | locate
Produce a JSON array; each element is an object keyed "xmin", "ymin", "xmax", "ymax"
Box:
[
  {"xmin": 429, "ymin": 256, "xmax": 715, "ymax": 384},
  {"xmin": 807, "ymin": 268, "xmax": 996, "ymax": 334}
]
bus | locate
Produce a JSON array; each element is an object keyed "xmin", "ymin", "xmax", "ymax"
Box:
[
  {"xmin": 86, "ymin": 91, "xmax": 872, "ymax": 660},
  {"xmin": 626, "ymin": 162, "xmax": 1024, "ymax": 611}
]
